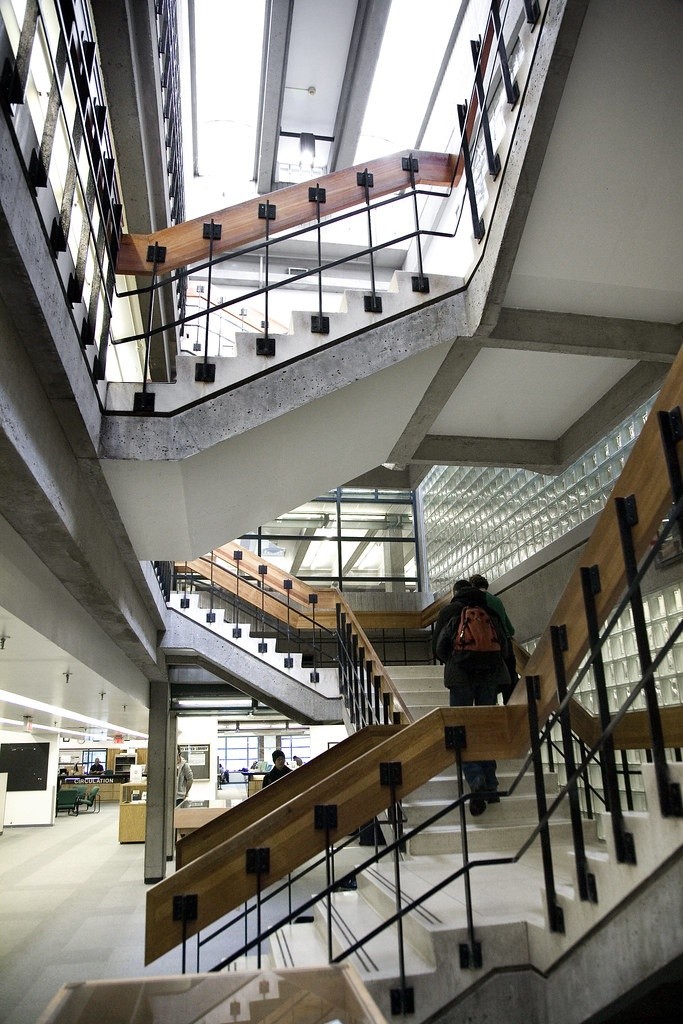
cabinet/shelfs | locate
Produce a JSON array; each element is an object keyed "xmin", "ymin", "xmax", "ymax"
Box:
[
  {"xmin": 113, "ymin": 754, "xmax": 137, "ymax": 782},
  {"xmin": 117, "ymin": 780, "xmax": 148, "ymax": 843}
]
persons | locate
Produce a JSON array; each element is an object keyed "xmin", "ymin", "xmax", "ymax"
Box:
[
  {"xmin": 89, "ymin": 757, "xmax": 104, "ymax": 775},
  {"xmin": 219, "ymin": 764, "xmax": 225, "ymax": 780},
  {"xmin": 176, "ymin": 744, "xmax": 193, "ymax": 839},
  {"xmin": 429, "ymin": 576, "xmax": 521, "ymax": 816},
  {"xmin": 293, "ymin": 756, "xmax": 302, "ymax": 766},
  {"xmin": 262, "ymin": 749, "xmax": 292, "ymax": 790}
]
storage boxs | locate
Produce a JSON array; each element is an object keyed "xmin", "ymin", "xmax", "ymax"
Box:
[{"xmin": 127, "ymin": 749, "xmax": 135, "ymax": 754}]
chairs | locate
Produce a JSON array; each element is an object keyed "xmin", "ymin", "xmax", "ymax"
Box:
[
  {"xmin": 56, "ymin": 786, "xmax": 101, "ymax": 817},
  {"xmin": 219, "ymin": 770, "xmax": 229, "ymax": 790}
]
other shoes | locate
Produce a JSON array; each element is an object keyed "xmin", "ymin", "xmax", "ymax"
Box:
[
  {"xmin": 487, "ymin": 786, "xmax": 500, "ymax": 804},
  {"xmin": 469, "ymin": 775, "xmax": 486, "ymax": 815}
]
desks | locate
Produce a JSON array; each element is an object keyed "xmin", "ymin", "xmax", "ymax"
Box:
[
  {"xmin": 57, "ymin": 774, "xmax": 130, "ymax": 803},
  {"xmin": 239, "ymin": 769, "xmax": 268, "ymax": 799}
]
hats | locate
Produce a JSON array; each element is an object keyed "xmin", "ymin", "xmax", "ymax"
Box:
[{"xmin": 272, "ymin": 750, "xmax": 285, "ymax": 763}]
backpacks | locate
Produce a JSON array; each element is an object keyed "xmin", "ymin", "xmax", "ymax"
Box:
[{"xmin": 449, "ymin": 600, "xmax": 503, "ymax": 667}]
